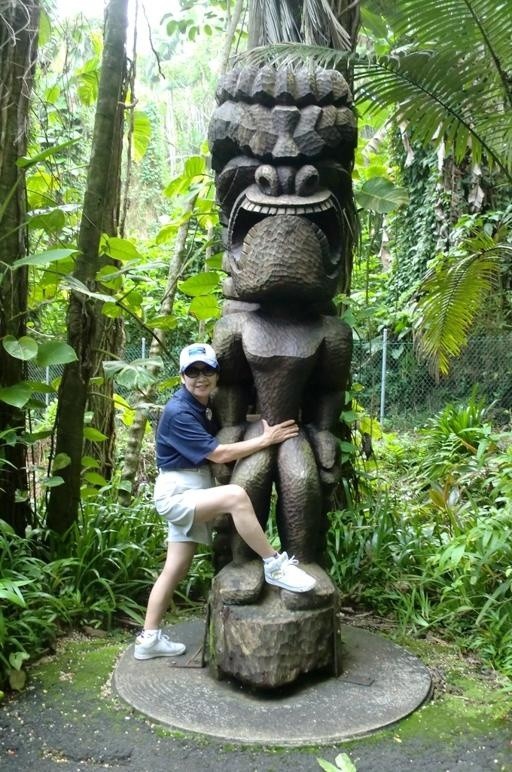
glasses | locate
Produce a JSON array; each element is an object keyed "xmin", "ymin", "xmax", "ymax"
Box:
[{"xmin": 184, "ymin": 365, "xmax": 218, "ymax": 379}]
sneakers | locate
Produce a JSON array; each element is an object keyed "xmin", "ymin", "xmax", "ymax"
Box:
[
  {"xmin": 135, "ymin": 631, "xmax": 186, "ymax": 660},
  {"xmin": 264, "ymin": 551, "xmax": 315, "ymax": 593}
]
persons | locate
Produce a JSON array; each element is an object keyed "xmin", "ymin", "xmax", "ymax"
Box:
[{"xmin": 132, "ymin": 341, "xmax": 319, "ymax": 664}]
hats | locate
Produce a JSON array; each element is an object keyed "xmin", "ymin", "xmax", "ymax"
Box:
[{"xmin": 179, "ymin": 343, "xmax": 219, "ymax": 369}]
